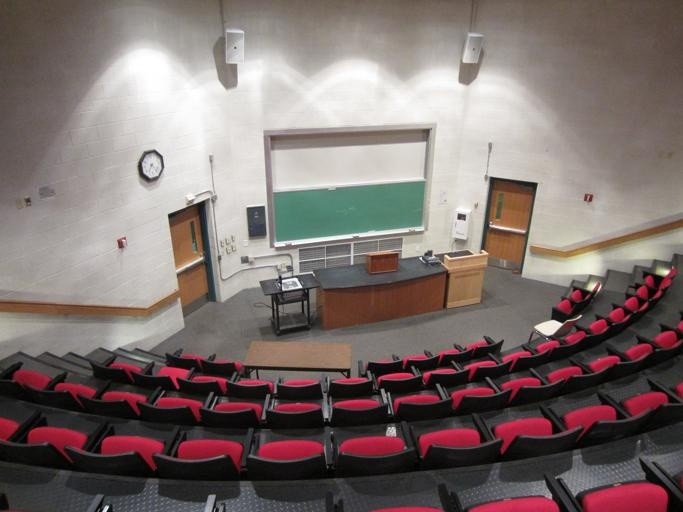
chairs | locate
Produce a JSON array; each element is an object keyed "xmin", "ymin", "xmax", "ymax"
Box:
[
  {"xmin": 515, "ymin": 265, "xmax": 683, "ymax": 508},
  {"xmin": 2, "ymin": 333, "xmax": 515, "ymax": 508}
]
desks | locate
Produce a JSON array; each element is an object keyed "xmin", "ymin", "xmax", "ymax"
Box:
[
  {"xmin": 257, "ymin": 274, "xmax": 321, "ymax": 335},
  {"xmin": 313, "ymin": 255, "xmax": 447, "ymax": 328}
]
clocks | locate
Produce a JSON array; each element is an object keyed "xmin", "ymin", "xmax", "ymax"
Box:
[{"xmin": 139, "ymin": 149, "xmax": 164, "ymax": 181}]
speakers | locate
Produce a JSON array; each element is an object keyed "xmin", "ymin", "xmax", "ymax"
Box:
[
  {"xmin": 461, "ymin": 33, "xmax": 484, "ymax": 64},
  {"xmin": 225, "ymin": 29, "xmax": 245, "ymax": 64}
]
